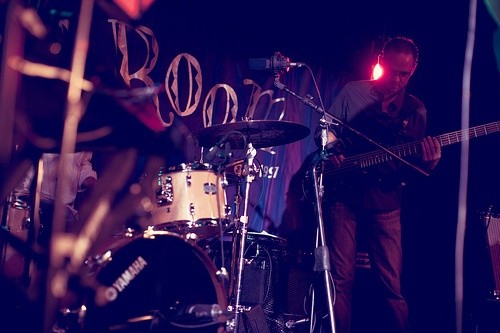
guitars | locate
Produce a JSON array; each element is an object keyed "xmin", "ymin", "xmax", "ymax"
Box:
[{"xmin": 301, "ymin": 117, "xmax": 500, "ymax": 206}]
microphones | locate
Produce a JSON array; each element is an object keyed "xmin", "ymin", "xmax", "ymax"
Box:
[
  {"xmin": 206, "ymin": 133, "xmax": 231, "ymax": 163},
  {"xmin": 248, "ymin": 58, "xmax": 304, "ymax": 70}
]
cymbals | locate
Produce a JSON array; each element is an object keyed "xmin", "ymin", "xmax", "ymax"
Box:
[{"xmin": 199, "ymin": 120, "xmax": 311, "ymax": 148}]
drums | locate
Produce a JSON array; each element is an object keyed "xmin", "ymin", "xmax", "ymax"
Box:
[
  {"xmin": 52, "ymin": 230, "xmax": 226, "ymax": 333},
  {"xmin": 214, "ymin": 232, "xmax": 287, "ymax": 306},
  {"xmin": 140, "ymin": 164, "xmax": 230, "ymax": 239},
  {"xmin": 3, "ymin": 193, "xmax": 77, "ymax": 285}
]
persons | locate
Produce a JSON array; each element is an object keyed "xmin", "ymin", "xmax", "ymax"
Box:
[
  {"xmin": 6, "ymin": 149, "xmax": 101, "ymax": 221},
  {"xmin": 313, "ymin": 37, "xmax": 441, "ymax": 333}
]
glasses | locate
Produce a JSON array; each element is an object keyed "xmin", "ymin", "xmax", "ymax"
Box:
[{"xmin": 381, "ymin": 62, "xmax": 412, "ymax": 77}]
